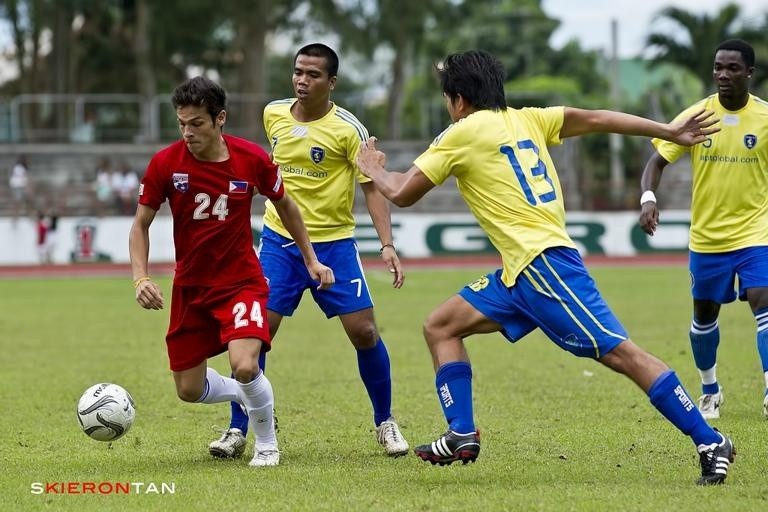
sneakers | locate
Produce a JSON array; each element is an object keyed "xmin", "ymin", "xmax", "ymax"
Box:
[
  {"xmin": 412, "ymin": 428, "xmax": 481, "ymax": 466},
  {"xmin": 697, "ymin": 384, "xmax": 725, "ymax": 420},
  {"xmin": 372, "ymin": 415, "xmax": 410, "ymax": 457},
  {"xmin": 695, "ymin": 426, "xmax": 737, "ymax": 486},
  {"xmin": 247, "ymin": 442, "xmax": 280, "ymax": 468},
  {"xmin": 209, "ymin": 426, "xmax": 248, "ymax": 459},
  {"xmin": 763, "ymin": 393, "xmax": 768, "ymax": 417}
]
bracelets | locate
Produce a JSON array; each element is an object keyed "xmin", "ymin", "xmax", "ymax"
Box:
[
  {"xmin": 381, "ymin": 244, "xmax": 395, "ymax": 251},
  {"xmin": 133, "ymin": 276, "xmax": 150, "ymax": 288},
  {"xmin": 640, "ymin": 191, "xmax": 657, "ymax": 206}
]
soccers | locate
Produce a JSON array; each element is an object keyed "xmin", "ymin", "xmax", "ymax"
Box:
[{"xmin": 77, "ymin": 382, "xmax": 137, "ymax": 441}]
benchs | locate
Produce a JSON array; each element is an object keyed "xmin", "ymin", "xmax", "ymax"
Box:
[{"xmin": 0, "ymin": 149, "xmax": 472, "ymax": 218}]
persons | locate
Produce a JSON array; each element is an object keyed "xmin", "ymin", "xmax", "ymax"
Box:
[
  {"xmin": 206, "ymin": 41, "xmax": 413, "ymax": 455},
  {"xmin": 639, "ymin": 39, "xmax": 768, "ymax": 422},
  {"xmin": 357, "ymin": 52, "xmax": 735, "ymax": 488},
  {"xmin": 128, "ymin": 77, "xmax": 335, "ymax": 463},
  {"xmin": 9, "ymin": 156, "xmax": 139, "ymax": 264}
]
